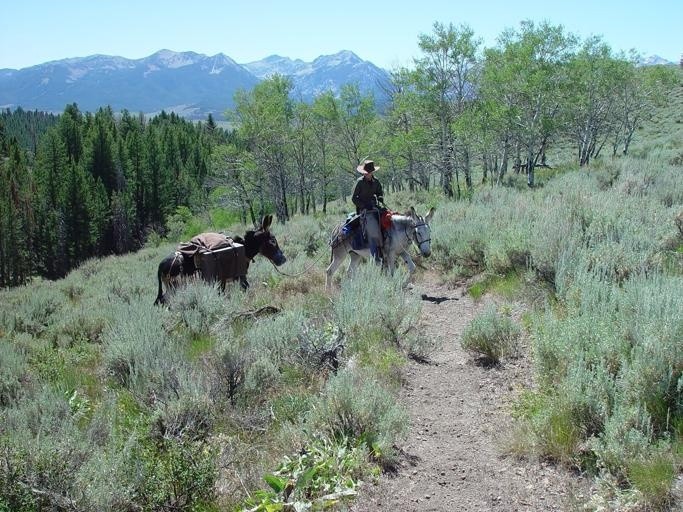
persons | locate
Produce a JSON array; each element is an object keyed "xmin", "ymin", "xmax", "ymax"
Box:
[{"xmin": 352, "ymin": 159, "xmax": 385, "ymax": 261}]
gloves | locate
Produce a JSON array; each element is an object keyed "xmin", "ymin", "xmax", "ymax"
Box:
[
  {"xmin": 377, "ymin": 196, "xmax": 383, "ymax": 202},
  {"xmin": 365, "ymin": 202, "xmax": 373, "ymax": 210}
]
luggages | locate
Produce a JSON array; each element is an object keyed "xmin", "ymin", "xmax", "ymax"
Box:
[{"xmin": 200, "ymin": 243, "xmax": 246, "ymax": 282}]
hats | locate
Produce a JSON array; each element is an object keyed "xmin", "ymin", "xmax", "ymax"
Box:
[{"xmin": 357, "ymin": 160, "xmax": 380, "ymax": 175}]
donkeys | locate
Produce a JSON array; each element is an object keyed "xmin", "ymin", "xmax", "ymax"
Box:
[
  {"xmin": 154, "ymin": 214, "xmax": 286, "ymax": 309},
  {"xmin": 325, "ymin": 206, "xmax": 435, "ymax": 292}
]
